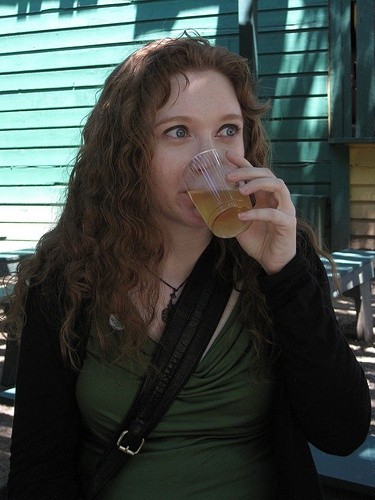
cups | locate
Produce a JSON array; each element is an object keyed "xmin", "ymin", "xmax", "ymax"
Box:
[{"xmin": 181, "ymin": 149, "xmax": 255, "ymax": 239}]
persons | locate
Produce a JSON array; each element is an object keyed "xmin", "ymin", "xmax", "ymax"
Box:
[{"xmin": 1, "ymin": 31, "xmax": 373, "ymax": 500}]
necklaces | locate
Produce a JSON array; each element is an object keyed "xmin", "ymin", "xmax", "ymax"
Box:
[{"xmin": 140, "ymin": 242, "xmax": 215, "ymax": 324}]
